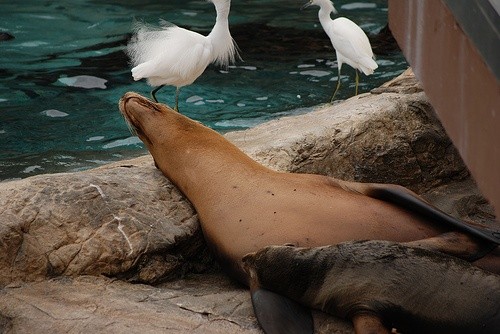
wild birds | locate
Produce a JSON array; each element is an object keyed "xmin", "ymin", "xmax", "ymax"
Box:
[
  {"xmin": 126, "ymin": 0, "xmax": 245, "ymax": 113},
  {"xmin": 303, "ymin": 0, "xmax": 378, "ymax": 103}
]
